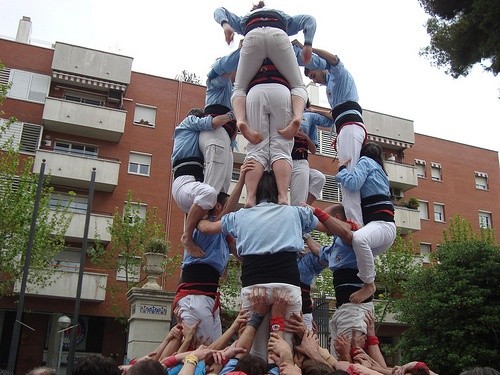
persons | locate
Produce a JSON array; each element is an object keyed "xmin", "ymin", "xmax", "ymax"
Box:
[
  {"xmin": 305, "ymin": 169, "xmax": 326, "ymax": 206},
  {"xmin": 176, "ymin": 193, "xmax": 231, "ymax": 348},
  {"xmin": 337, "ymin": 143, "xmax": 396, "ymax": 303},
  {"xmin": 289, "ymin": 39, "xmax": 366, "ymax": 229},
  {"xmin": 209, "ymin": 47, "xmax": 331, "ymax": 208},
  {"xmin": 214, "ymin": 3, "xmax": 318, "ymax": 144},
  {"xmin": 289, "ymin": 100, "xmax": 335, "ymax": 206},
  {"xmin": 199, "ymin": 39, "xmax": 245, "ymax": 214},
  {"xmin": 171, "ymin": 108, "xmax": 233, "ymax": 257},
  {"xmin": 26, "ymin": 287, "xmax": 498, "ymax": 375},
  {"xmin": 196, "ymin": 172, "xmax": 360, "ymax": 362},
  {"xmin": 303, "ymin": 204, "xmax": 374, "ymax": 360}
]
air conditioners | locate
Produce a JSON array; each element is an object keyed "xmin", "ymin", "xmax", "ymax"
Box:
[
  {"xmin": 42, "ymin": 140, "xmax": 52, "ymax": 146},
  {"xmin": 392, "ymin": 188, "xmax": 405, "ymax": 197},
  {"xmin": 107, "ymin": 89, "xmax": 122, "ymax": 103}
]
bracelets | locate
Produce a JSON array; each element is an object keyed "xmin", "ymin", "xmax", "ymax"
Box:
[{"xmin": 184, "ymin": 355, "xmax": 199, "ymax": 366}]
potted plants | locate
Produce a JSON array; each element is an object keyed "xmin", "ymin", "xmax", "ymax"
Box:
[{"xmin": 142, "ymin": 238, "xmax": 169, "ymax": 266}]
